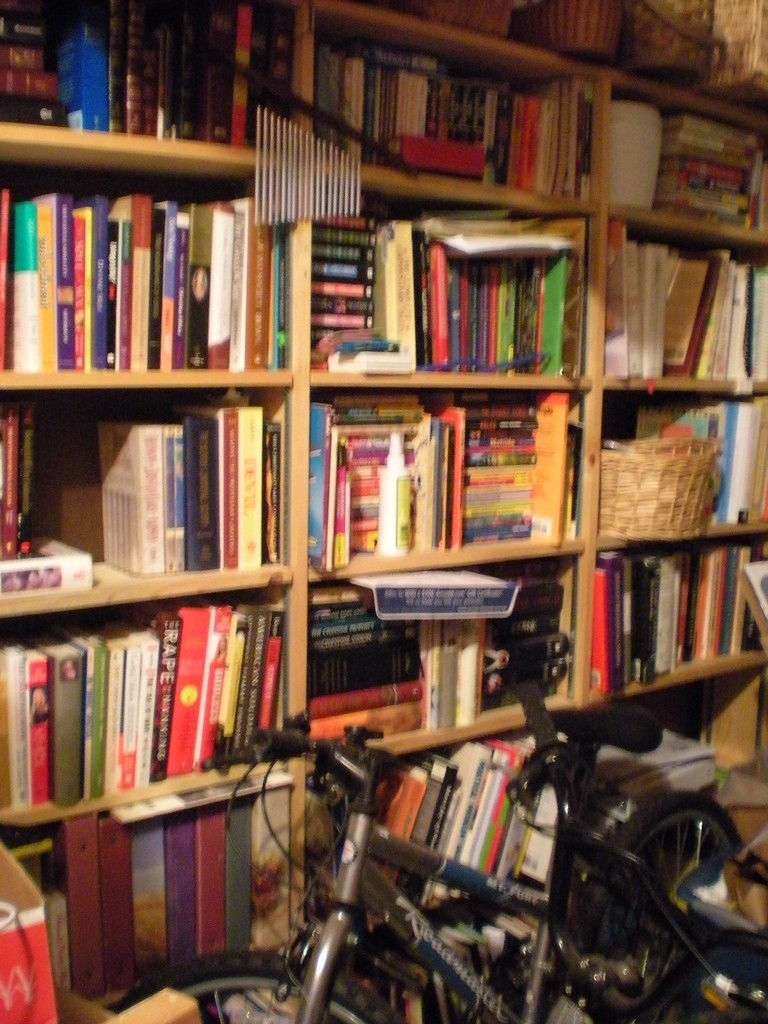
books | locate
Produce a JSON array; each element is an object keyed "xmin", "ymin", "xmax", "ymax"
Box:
[{"xmin": 0, "ymin": 0, "xmax": 768, "ymax": 1001}]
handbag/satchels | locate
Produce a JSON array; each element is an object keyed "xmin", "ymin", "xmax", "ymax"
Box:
[{"xmin": 724, "ymin": 839, "xmax": 768, "ymax": 931}]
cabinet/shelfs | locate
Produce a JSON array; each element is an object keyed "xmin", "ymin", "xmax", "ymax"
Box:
[
  {"xmin": 585, "ymin": 68, "xmax": 768, "ymax": 861},
  {"xmin": 301, "ymin": 0, "xmax": 589, "ymax": 968},
  {"xmin": 0, "ymin": 1, "xmax": 298, "ymax": 1024}
]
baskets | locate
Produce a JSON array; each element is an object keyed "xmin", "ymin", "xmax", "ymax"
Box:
[{"xmin": 599, "ymin": 439, "xmax": 719, "ymax": 541}]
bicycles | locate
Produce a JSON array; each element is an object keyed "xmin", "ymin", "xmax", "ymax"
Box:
[{"xmin": 117, "ymin": 710, "xmax": 768, "ymax": 1023}]
